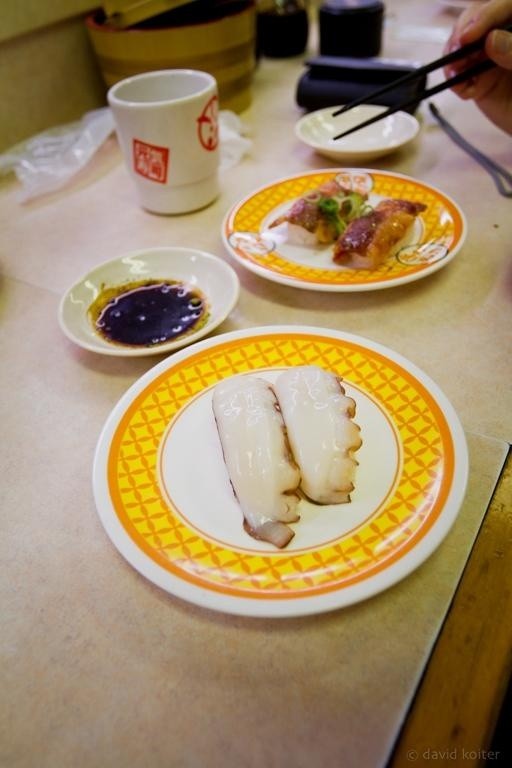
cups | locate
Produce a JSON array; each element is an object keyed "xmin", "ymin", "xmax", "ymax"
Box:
[
  {"xmin": 318, "ymin": 0, "xmax": 383, "ymax": 64},
  {"xmin": 106, "ymin": 69, "xmax": 220, "ymax": 217},
  {"xmin": 256, "ymin": 0, "xmax": 310, "ymax": 57}
]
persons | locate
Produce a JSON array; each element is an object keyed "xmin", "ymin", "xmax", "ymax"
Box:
[{"xmin": 443, "ymin": 1, "xmax": 512, "ymax": 135}]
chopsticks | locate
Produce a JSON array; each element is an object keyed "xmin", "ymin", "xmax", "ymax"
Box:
[{"xmin": 331, "ymin": 25, "xmax": 511, "ymax": 141}]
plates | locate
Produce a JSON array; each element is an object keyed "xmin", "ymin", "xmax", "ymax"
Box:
[
  {"xmin": 58, "ymin": 246, "xmax": 241, "ymax": 358},
  {"xmin": 91, "ymin": 324, "xmax": 471, "ymax": 619},
  {"xmin": 222, "ymin": 168, "xmax": 468, "ymax": 293},
  {"xmin": 294, "ymin": 104, "xmax": 418, "ymax": 162}
]
